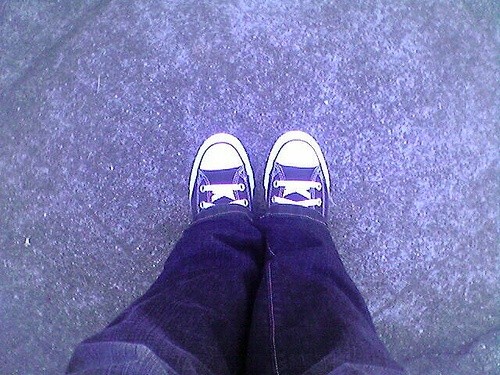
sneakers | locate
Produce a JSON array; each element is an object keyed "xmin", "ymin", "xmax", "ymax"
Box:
[
  {"xmin": 264, "ymin": 130, "xmax": 331, "ymax": 227},
  {"xmin": 187, "ymin": 133, "xmax": 255, "ymax": 221}
]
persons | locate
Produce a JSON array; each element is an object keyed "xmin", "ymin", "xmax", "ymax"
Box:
[{"xmin": 61, "ymin": 128, "xmax": 412, "ymax": 375}]
